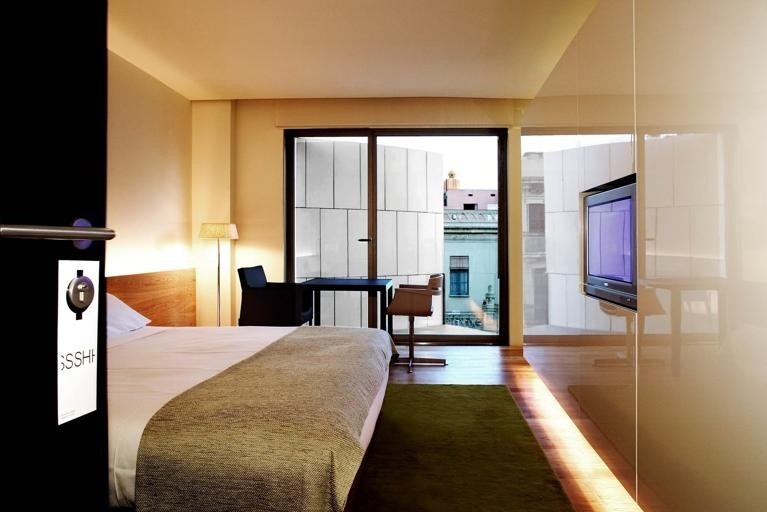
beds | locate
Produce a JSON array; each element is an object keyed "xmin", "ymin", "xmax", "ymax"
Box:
[{"xmin": 105, "ymin": 325, "xmax": 400, "ymax": 510}]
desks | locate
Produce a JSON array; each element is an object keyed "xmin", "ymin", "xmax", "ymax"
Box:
[{"xmin": 298, "ymin": 276, "xmax": 394, "ymax": 340}]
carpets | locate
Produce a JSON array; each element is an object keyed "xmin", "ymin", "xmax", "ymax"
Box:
[{"xmin": 343, "ymin": 383, "xmax": 576, "ymax": 510}]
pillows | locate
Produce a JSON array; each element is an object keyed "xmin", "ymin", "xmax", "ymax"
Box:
[{"xmin": 106, "ymin": 286, "xmax": 155, "ymax": 341}]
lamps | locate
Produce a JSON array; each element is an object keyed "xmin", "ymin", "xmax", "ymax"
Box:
[{"xmin": 199, "ymin": 222, "xmax": 239, "ymax": 327}]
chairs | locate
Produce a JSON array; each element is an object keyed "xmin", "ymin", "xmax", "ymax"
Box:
[
  {"xmin": 384, "ymin": 272, "xmax": 447, "ymax": 373},
  {"xmin": 237, "ymin": 265, "xmax": 314, "ymax": 327}
]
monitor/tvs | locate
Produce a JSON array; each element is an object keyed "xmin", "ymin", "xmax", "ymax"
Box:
[{"xmin": 580, "ymin": 183, "xmax": 639, "ymax": 311}]
